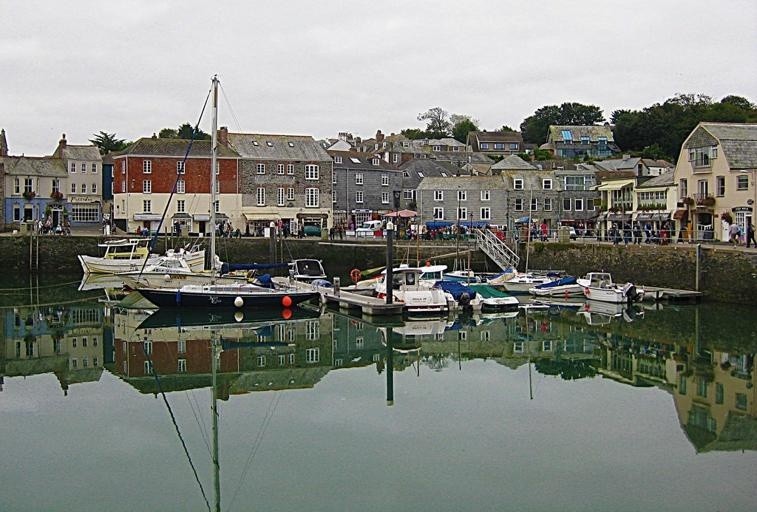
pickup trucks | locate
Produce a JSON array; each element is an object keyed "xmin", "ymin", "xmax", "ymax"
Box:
[{"xmin": 356, "ymin": 220, "xmax": 387, "ymax": 236}]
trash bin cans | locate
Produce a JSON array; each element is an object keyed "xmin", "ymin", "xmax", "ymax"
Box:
[
  {"xmin": 180, "ymin": 224, "xmax": 189, "ymax": 238},
  {"xmin": 320, "ymin": 227, "xmax": 329, "ymax": 240},
  {"xmin": 20, "ymin": 223, "xmax": 27, "ymax": 235}
]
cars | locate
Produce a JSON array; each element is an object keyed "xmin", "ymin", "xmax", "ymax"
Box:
[{"xmin": 560, "ymin": 226, "xmax": 578, "ymax": 241}]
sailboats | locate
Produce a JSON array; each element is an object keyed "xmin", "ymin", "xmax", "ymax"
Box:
[{"xmin": 134, "ymin": 308, "xmax": 321, "ymax": 512}]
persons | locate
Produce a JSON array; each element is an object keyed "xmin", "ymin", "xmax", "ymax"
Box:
[
  {"xmin": 404, "ymin": 223, "xmax": 467, "ymax": 242},
  {"xmin": 136, "ymin": 226, "xmax": 149, "ymax": 237},
  {"xmin": 38, "ymin": 220, "xmax": 71, "ymax": 236},
  {"xmin": 495, "ymin": 222, "xmax": 551, "ymax": 241},
  {"xmin": 256, "ymin": 222, "xmax": 343, "ymax": 241},
  {"xmin": 609, "ymin": 222, "xmax": 671, "ymax": 248},
  {"xmin": 215, "ymin": 221, "xmax": 234, "ymax": 238},
  {"xmin": 728, "ymin": 219, "xmax": 757, "ymax": 249}
]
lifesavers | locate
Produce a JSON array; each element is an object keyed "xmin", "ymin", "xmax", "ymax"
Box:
[{"xmin": 350, "ymin": 269, "xmax": 361, "ymax": 281}]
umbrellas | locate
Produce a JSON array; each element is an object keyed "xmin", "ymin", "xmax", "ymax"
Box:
[{"xmin": 383, "ymin": 209, "xmax": 417, "ymax": 227}]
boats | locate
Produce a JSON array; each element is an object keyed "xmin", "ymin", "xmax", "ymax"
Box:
[{"xmin": 376, "ymin": 291, "xmax": 649, "ymax": 354}]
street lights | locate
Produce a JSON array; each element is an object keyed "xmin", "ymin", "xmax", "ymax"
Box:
[
  {"xmin": 469, "ymin": 213, "xmax": 474, "ymax": 239},
  {"xmin": 433, "ymin": 214, "xmax": 438, "ymax": 239}
]
